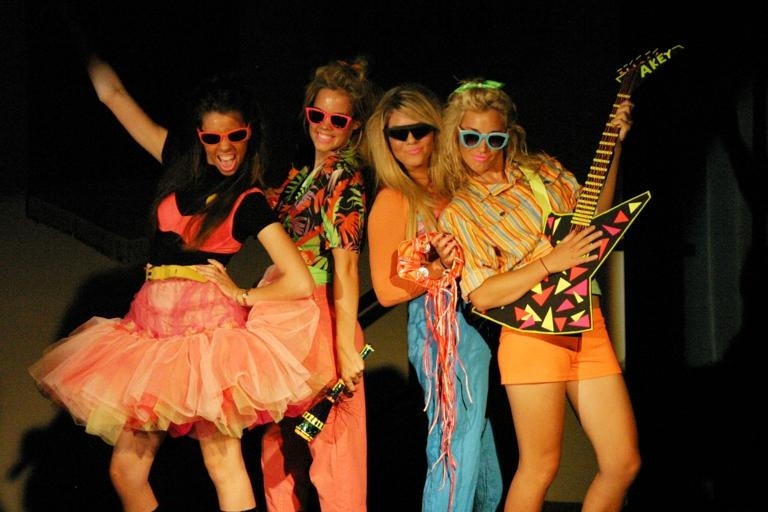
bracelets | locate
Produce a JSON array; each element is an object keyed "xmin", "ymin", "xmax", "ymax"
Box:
[
  {"xmin": 539, "ymin": 258, "xmax": 551, "ymax": 276},
  {"xmin": 439, "ymin": 258, "xmax": 448, "ymax": 270}
]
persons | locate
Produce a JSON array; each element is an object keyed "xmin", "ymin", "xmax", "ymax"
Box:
[
  {"xmin": 363, "ymin": 84, "xmax": 504, "ymax": 512},
  {"xmin": 256, "ymin": 59, "xmax": 375, "ymax": 511},
  {"xmin": 27, "ymin": 56, "xmax": 315, "ymax": 510},
  {"xmin": 443, "ymin": 80, "xmax": 637, "ymax": 511}
]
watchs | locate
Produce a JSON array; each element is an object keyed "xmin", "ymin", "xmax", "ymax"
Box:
[{"xmin": 242, "ymin": 288, "xmax": 250, "ymax": 307}]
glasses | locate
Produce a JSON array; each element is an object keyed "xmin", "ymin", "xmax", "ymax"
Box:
[
  {"xmin": 382, "ymin": 122, "xmax": 437, "ymax": 142},
  {"xmin": 457, "ymin": 125, "xmax": 511, "ymax": 151},
  {"xmin": 305, "ymin": 107, "xmax": 354, "ymax": 132},
  {"xmin": 195, "ymin": 122, "xmax": 252, "ymax": 147}
]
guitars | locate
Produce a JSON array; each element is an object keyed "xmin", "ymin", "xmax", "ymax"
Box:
[{"xmin": 470, "ymin": 41, "xmax": 684, "ymax": 336}]
